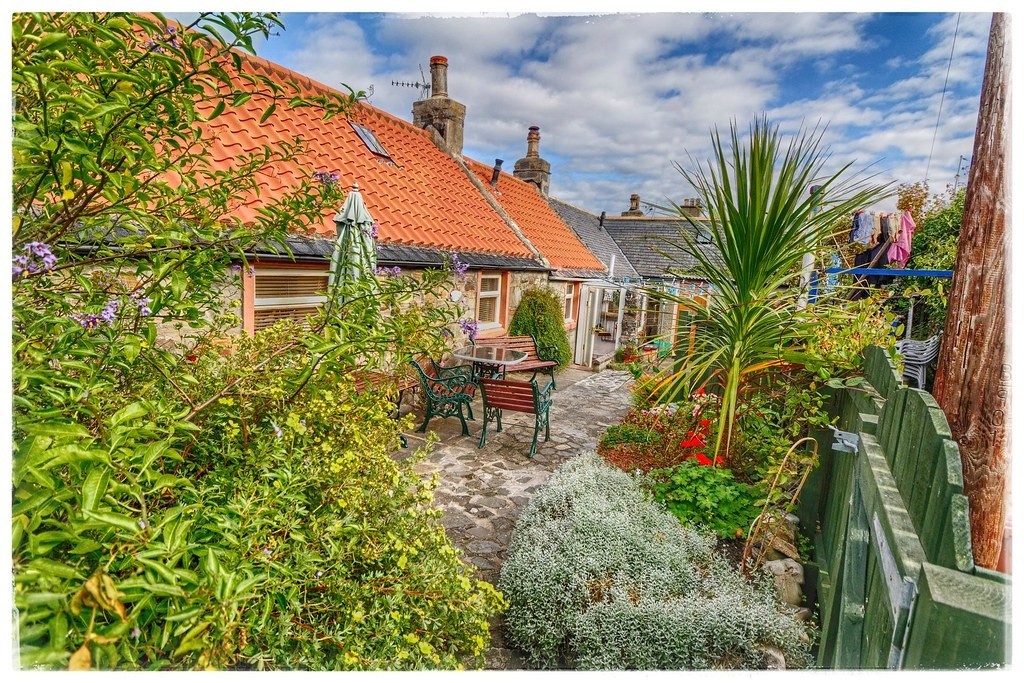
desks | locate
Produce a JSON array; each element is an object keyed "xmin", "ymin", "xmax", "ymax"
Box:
[{"xmin": 452, "ymin": 346, "xmax": 528, "ymax": 419}]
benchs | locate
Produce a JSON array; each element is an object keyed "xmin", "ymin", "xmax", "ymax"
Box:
[
  {"xmin": 470, "ymin": 335, "xmax": 561, "ymax": 402},
  {"xmin": 344, "ymin": 362, "xmax": 417, "ymax": 435},
  {"xmin": 478, "ymin": 377, "xmax": 554, "ymax": 458}
]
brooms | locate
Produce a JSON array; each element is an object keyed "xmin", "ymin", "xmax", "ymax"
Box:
[{"xmin": 594, "ymin": 301, "xmax": 612, "ymax": 337}]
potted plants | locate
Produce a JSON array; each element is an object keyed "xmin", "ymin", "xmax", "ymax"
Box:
[{"xmin": 621, "ymin": 340, "xmax": 636, "ymax": 362}]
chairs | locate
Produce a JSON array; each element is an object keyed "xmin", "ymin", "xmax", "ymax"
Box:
[{"xmin": 408, "ymin": 351, "xmax": 477, "ymax": 437}]
